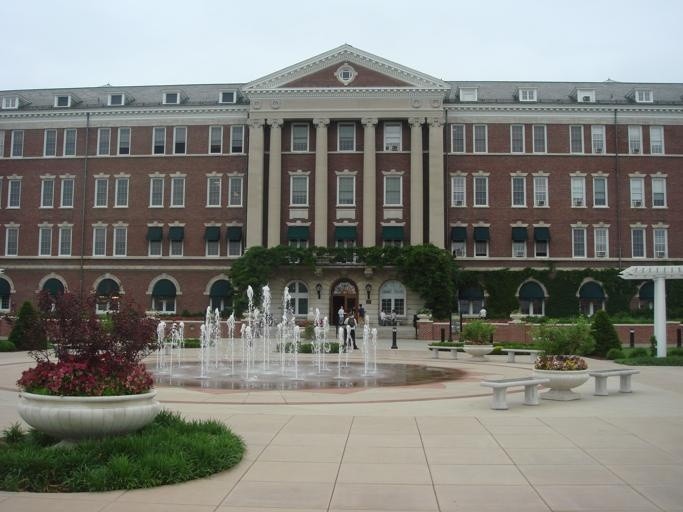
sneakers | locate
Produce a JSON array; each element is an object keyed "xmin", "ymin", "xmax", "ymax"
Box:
[{"xmin": 354, "ymin": 347, "xmax": 359, "ymax": 349}]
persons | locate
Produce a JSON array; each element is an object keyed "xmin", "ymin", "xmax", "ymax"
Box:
[
  {"xmin": 390, "ymin": 309, "xmax": 396, "ymax": 325},
  {"xmin": 350, "ymin": 305, "xmax": 356, "ymax": 315},
  {"xmin": 379, "ymin": 310, "xmax": 390, "ymax": 325},
  {"xmin": 338, "ymin": 306, "xmax": 344, "ymax": 327},
  {"xmin": 344, "ymin": 313, "xmax": 361, "ymax": 345},
  {"xmin": 358, "ymin": 304, "xmax": 367, "ymax": 322}
]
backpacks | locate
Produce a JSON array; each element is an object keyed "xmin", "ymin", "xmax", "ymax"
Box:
[{"xmin": 348, "ymin": 318, "xmax": 354, "ymax": 327}]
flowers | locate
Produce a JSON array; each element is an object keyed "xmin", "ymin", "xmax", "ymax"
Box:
[
  {"xmin": 16, "ymin": 357, "xmax": 153, "ymax": 394},
  {"xmin": 535, "ymin": 354, "xmax": 587, "ymax": 371}
]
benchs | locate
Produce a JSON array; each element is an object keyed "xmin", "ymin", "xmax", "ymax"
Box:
[
  {"xmin": 481, "ymin": 368, "xmax": 639, "ymax": 410},
  {"xmin": 427, "ymin": 346, "xmax": 463, "ymax": 360},
  {"xmin": 500, "ymin": 348, "xmax": 546, "ymax": 364}
]
